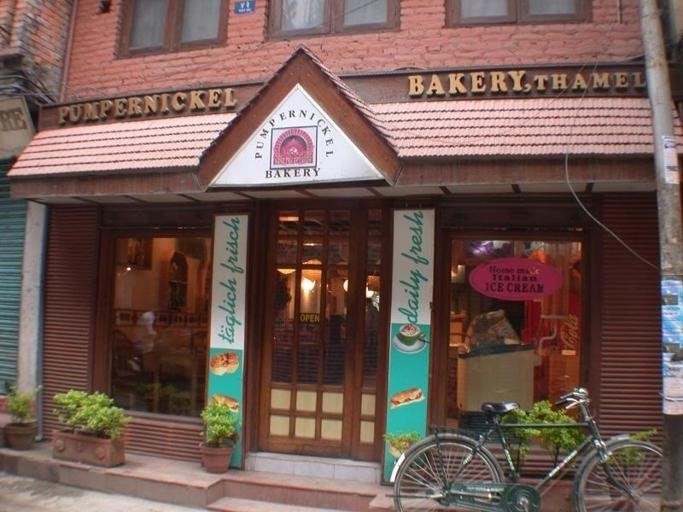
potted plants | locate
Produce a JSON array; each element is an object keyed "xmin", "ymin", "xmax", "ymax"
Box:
[
  {"xmin": 603, "ymin": 429, "xmax": 658, "ymax": 512},
  {"xmin": 506, "ymin": 401, "xmax": 589, "ymax": 508},
  {"xmin": 383, "ymin": 433, "xmax": 432, "ymax": 492},
  {"xmin": 4, "ymin": 384, "xmax": 44, "ymax": 450},
  {"xmin": 51, "ymin": 390, "xmax": 132, "ymax": 467},
  {"xmin": 199, "ymin": 400, "xmax": 238, "ymax": 473}
]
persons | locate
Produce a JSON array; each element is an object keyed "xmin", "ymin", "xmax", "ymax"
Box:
[{"xmin": 367, "ymin": 297, "xmax": 378, "ymax": 363}]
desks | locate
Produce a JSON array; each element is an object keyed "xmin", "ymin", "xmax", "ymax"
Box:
[{"xmin": 153, "ymin": 356, "xmax": 206, "ymax": 418}]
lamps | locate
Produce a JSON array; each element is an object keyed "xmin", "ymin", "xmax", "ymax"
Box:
[{"xmin": 301, "ymin": 279, "xmax": 316, "ymax": 292}]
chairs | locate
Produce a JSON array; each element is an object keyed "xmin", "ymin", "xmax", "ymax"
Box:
[
  {"xmin": 112, "ymin": 331, "xmax": 154, "ymax": 413},
  {"xmin": 191, "ymin": 330, "xmax": 208, "ymax": 358}
]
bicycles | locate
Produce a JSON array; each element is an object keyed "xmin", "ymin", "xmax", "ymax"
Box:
[{"xmin": 389, "ymin": 386, "xmax": 662, "ymax": 511}]
